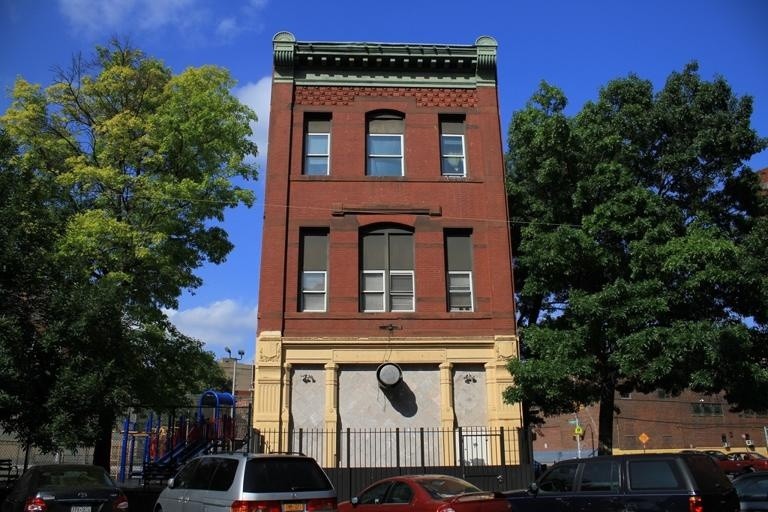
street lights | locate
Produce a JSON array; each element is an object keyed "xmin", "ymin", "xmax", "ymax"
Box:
[{"xmin": 225, "ymin": 344, "xmax": 245, "ymax": 419}]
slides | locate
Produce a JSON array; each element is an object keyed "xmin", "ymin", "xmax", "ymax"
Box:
[{"xmin": 148, "ymin": 441, "xmax": 214, "ymax": 472}]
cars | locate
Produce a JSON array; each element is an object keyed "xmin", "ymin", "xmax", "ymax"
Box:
[
  {"xmin": 727, "ymin": 453, "xmax": 767, "ymax": 473},
  {"xmin": 729, "ymin": 470, "xmax": 767, "ymax": 511},
  {"xmin": 0, "ymin": 464, "xmax": 130, "ymax": 512},
  {"xmin": 335, "ymin": 475, "xmax": 511, "ymax": 511},
  {"xmin": 703, "ymin": 451, "xmax": 754, "ymax": 475}
]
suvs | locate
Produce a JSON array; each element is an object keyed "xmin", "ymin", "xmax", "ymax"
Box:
[{"xmin": 494, "ymin": 452, "xmax": 741, "ymax": 511}]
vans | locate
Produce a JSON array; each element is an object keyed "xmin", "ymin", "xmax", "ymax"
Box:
[{"xmin": 150, "ymin": 449, "xmax": 338, "ymax": 511}]
